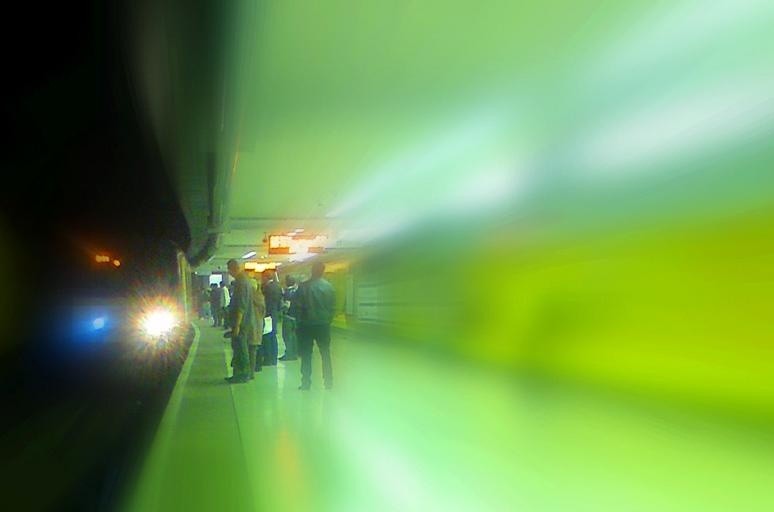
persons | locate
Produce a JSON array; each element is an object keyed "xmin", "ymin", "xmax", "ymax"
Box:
[{"xmin": 196, "ymin": 259, "xmax": 336, "ymax": 390}]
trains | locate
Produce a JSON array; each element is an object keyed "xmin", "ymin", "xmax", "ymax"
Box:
[{"xmin": 44, "ymin": 230, "xmax": 196, "ymax": 378}]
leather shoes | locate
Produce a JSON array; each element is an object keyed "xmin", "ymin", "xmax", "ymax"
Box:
[
  {"xmin": 279, "ymin": 355, "xmax": 297, "ymax": 360},
  {"xmin": 225, "ymin": 375, "xmax": 254, "ymax": 383}
]
